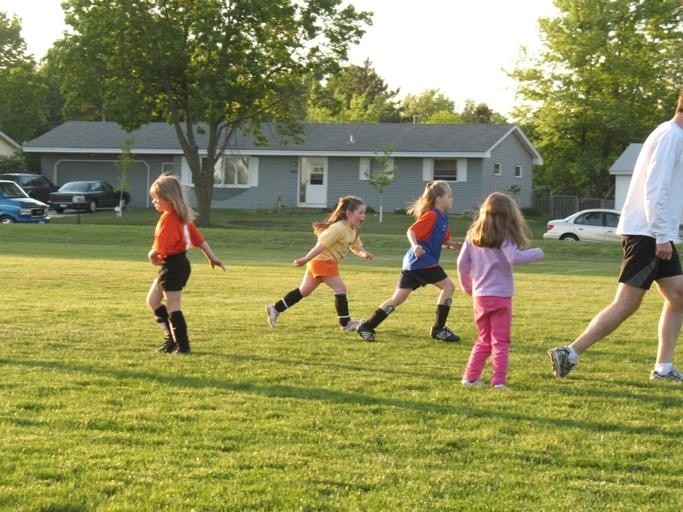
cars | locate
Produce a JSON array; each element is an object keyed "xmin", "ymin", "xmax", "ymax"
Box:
[
  {"xmin": 1, "ymin": 172, "xmax": 61, "ymax": 215},
  {"xmin": 0, "ymin": 180, "xmax": 51, "ymax": 225},
  {"xmin": 541, "ymin": 206, "xmax": 681, "ymax": 245},
  {"xmin": 49, "ymin": 177, "xmax": 131, "ymax": 216}
]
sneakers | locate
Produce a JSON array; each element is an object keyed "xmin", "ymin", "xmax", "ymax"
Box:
[
  {"xmin": 430, "ymin": 326, "xmax": 459, "ymax": 342},
  {"xmin": 492, "ymin": 383, "xmax": 506, "ymax": 390},
  {"xmin": 340, "ymin": 319, "xmax": 363, "ymax": 332},
  {"xmin": 461, "ymin": 379, "xmax": 487, "ymax": 388},
  {"xmin": 649, "ymin": 367, "xmax": 683, "ymax": 382},
  {"xmin": 155, "ymin": 340, "xmax": 176, "ymax": 353},
  {"xmin": 265, "ymin": 304, "xmax": 280, "ymax": 328},
  {"xmin": 171, "ymin": 346, "xmax": 190, "ymax": 354},
  {"xmin": 357, "ymin": 322, "xmax": 375, "ymax": 342},
  {"xmin": 546, "ymin": 345, "xmax": 576, "ymax": 380}
]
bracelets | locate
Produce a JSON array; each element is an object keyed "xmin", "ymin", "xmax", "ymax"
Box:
[{"xmin": 456, "ymin": 241, "xmax": 463, "ymax": 251}]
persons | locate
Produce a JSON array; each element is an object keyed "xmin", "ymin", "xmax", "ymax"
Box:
[
  {"xmin": 545, "ymin": 89, "xmax": 683, "ymax": 381},
  {"xmin": 357, "ymin": 179, "xmax": 464, "ymax": 341},
  {"xmin": 264, "ymin": 195, "xmax": 374, "ymax": 331},
  {"xmin": 454, "ymin": 191, "xmax": 545, "ymax": 388},
  {"xmin": 143, "ymin": 171, "xmax": 226, "ymax": 359}
]
